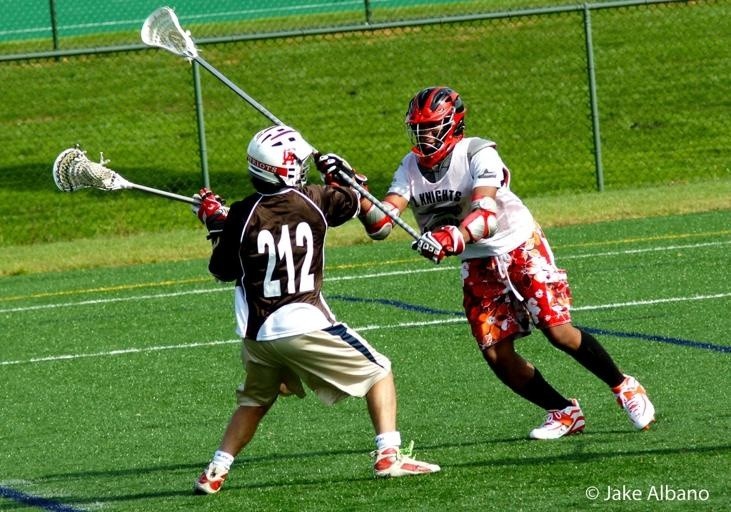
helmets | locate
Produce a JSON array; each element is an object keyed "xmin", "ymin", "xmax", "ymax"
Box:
[
  {"xmin": 247, "ymin": 125, "xmax": 313, "ymax": 188},
  {"xmin": 405, "ymin": 87, "xmax": 466, "ymax": 169}
]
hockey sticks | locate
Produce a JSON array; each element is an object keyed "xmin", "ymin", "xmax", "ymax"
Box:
[
  {"xmin": 140, "ymin": 7, "xmax": 421, "ymax": 240},
  {"xmin": 53, "ymin": 144, "xmax": 231, "ymax": 214}
]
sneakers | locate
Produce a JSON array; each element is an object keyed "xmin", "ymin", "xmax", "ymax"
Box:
[
  {"xmin": 370, "ymin": 440, "xmax": 441, "ymax": 477},
  {"xmin": 529, "ymin": 397, "xmax": 585, "ymax": 440},
  {"xmin": 196, "ymin": 465, "xmax": 228, "ymax": 494},
  {"xmin": 611, "ymin": 374, "xmax": 657, "ymax": 431}
]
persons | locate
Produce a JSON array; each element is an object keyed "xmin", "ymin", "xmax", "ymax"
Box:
[
  {"xmin": 193, "ymin": 124, "xmax": 442, "ymax": 495},
  {"xmin": 312, "ymin": 86, "xmax": 656, "ymax": 441}
]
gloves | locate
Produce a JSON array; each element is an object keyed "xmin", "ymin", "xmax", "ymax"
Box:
[
  {"xmin": 319, "ymin": 152, "xmax": 355, "ymax": 186},
  {"xmin": 192, "ymin": 188, "xmax": 228, "ymax": 240},
  {"xmin": 411, "ymin": 225, "xmax": 465, "ymax": 264}
]
